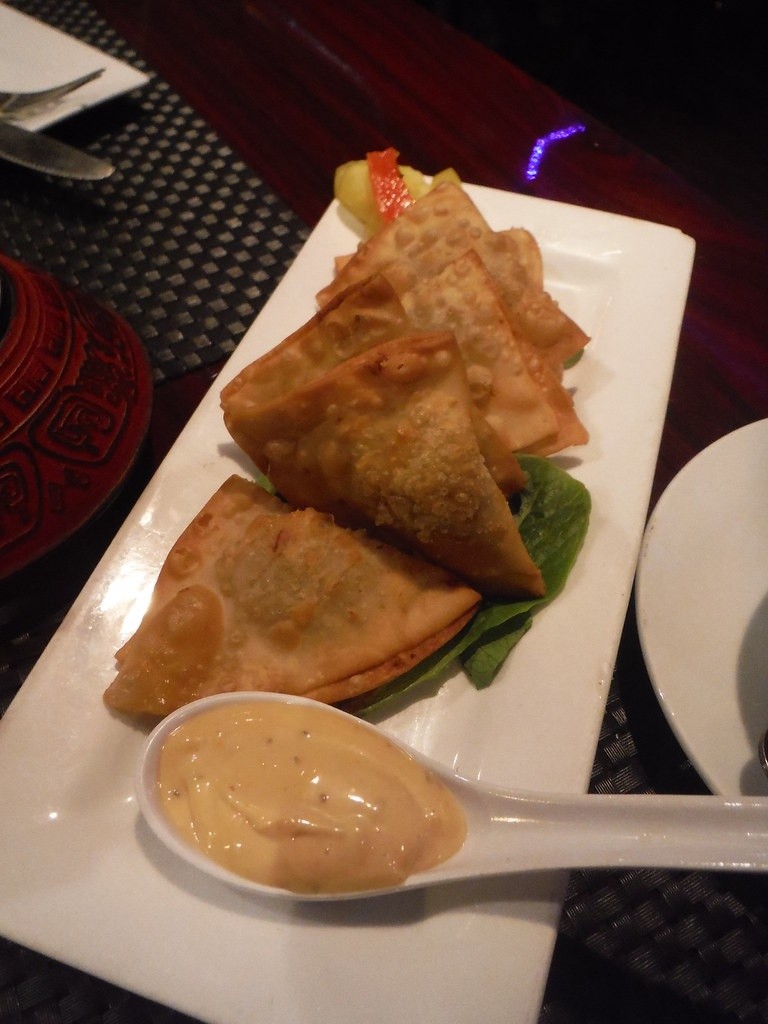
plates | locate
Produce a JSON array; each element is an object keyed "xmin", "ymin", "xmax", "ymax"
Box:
[
  {"xmin": 0, "ymin": 0, "xmax": 151, "ymax": 133},
  {"xmin": 635, "ymin": 417, "xmax": 768, "ymax": 796},
  {"xmin": 0, "ymin": 177, "xmax": 696, "ymax": 1024}
]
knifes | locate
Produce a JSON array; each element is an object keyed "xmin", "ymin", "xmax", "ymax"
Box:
[{"xmin": 0, "ymin": 119, "xmax": 118, "ymax": 181}]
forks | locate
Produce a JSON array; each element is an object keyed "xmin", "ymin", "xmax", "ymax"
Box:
[{"xmin": 0, "ymin": 68, "xmax": 107, "ymax": 119}]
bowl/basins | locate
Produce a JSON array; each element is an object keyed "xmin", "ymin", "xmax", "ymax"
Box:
[{"xmin": 0, "ymin": 255, "xmax": 155, "ymax": 577}]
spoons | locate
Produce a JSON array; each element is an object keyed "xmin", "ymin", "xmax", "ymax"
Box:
[{"xmin": 136, "ymin": 691, "xmax": 768, "ymax": 902}]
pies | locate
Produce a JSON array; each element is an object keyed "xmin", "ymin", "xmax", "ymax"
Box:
[{"xmin": 105, "ymin": 171, "xmax": 587, "ymax": 716}]
